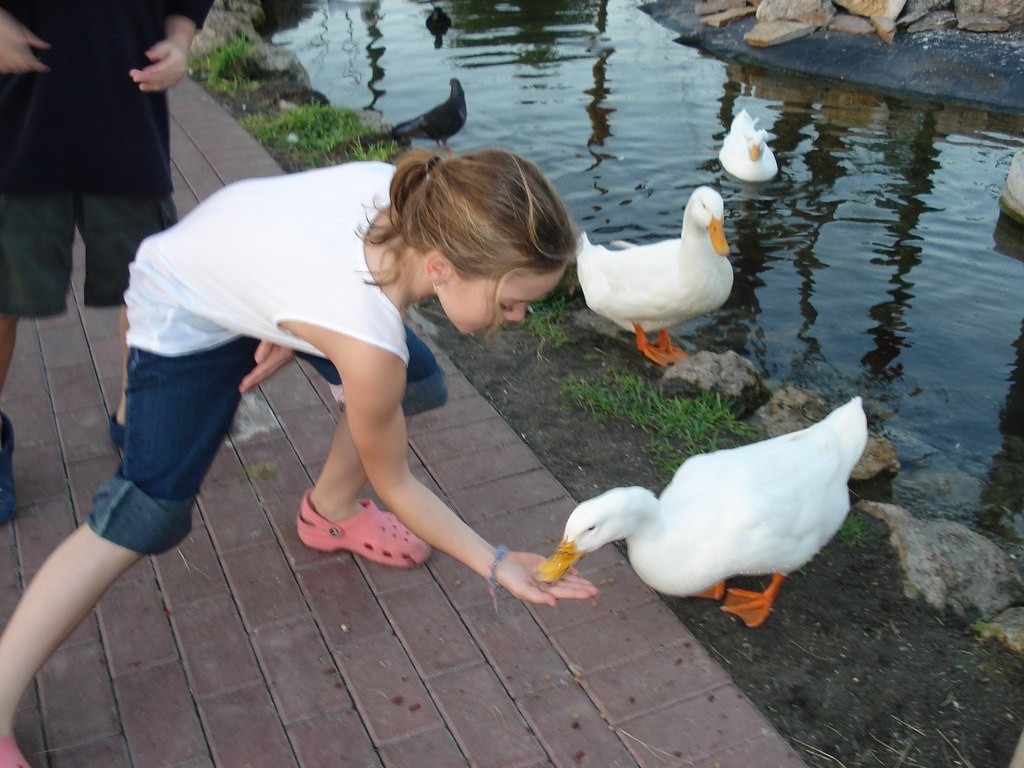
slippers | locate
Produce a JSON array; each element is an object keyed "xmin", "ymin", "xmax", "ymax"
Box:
[
  {"xmin": 0, "ymin": 410, "xmax": 16, "ymax": 524},
  {"xmin": 109, "ymin": 412, "xmax": 125, "ymax": 446}
]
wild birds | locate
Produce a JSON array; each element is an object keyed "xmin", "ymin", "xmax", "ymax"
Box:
[
  {"xmin": 389, "ymin": 78, "xmax": 467, "ymax": 152},
  {"xmin": 426, "ymin": 7, "xmax": 451, "ymax": 33}
]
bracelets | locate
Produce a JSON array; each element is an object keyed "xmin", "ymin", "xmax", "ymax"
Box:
[{"xmin": 488, "ymin": 545, "xmax": 509, "ymax": 616}]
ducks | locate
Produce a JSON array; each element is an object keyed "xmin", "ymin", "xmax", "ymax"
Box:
[
  {"xmin": 534, "ymin": 397, "xmax": 868, "ymax": 628},
  {"xmin": 718, "ymin": 108, "xmax": 778, "ymax": 182},
  {"xmin": 576, "ymin": 186, "xmax": 734, "ymax": 367}
]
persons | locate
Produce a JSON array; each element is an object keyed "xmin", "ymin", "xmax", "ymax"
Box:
[
  {"xmin": 1, "ymin": 145, "xmax": 598, "ymax": 768},
  {"xmin": 0, "ymin": 0, "xmax": 215, "ymax": 517}
]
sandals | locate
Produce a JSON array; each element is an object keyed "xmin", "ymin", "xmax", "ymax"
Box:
[
  {"xmin": 0, "ymin": 734, "xmax": 33, "ymax": 768},
  {"xmin": 297, "ymin": 488, "xmax": 432, "ymax": 567}
]
